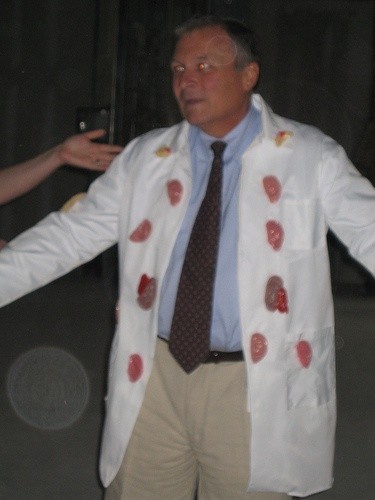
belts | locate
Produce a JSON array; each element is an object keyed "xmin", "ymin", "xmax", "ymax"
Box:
[{"xmin": 207, "ymin": 351, "xmax": 244, "ymax": 362}]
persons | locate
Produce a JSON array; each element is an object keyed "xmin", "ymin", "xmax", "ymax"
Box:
[
  {"xmin": 1, "ymin": 15, "xmax": 375, "ymax": 499},
  {"xmin": 1, "ymin": 128, "xmax": 123, "ymax": 206}
]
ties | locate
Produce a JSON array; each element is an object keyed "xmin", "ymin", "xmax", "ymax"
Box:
[{"xmin": 169, "ymin": 141, "xmax": 228, "ymax": 375}]
